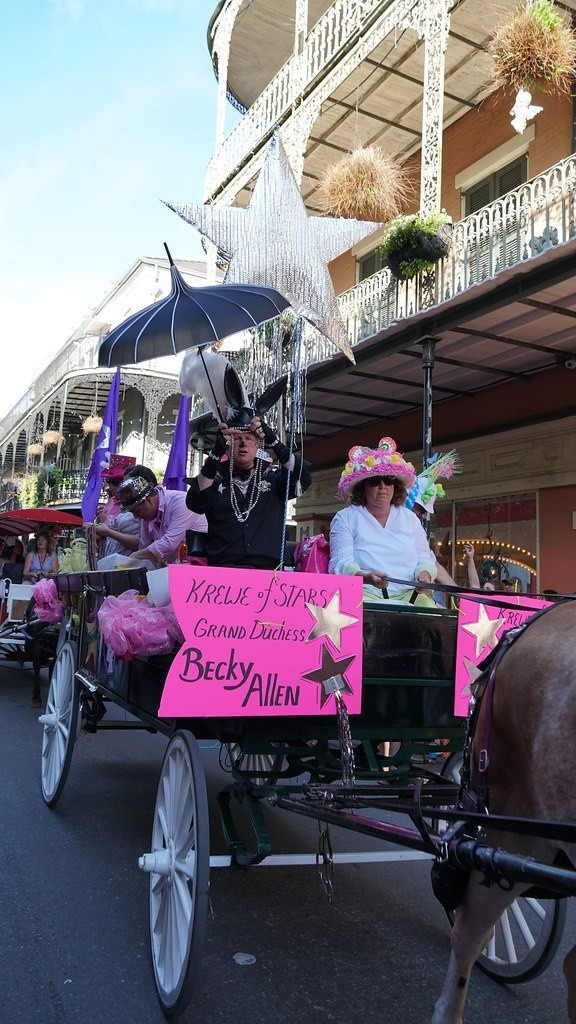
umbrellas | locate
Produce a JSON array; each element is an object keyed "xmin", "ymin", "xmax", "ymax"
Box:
[{"xmin": 98, "ymin": 239, "xmax": 291, "ymax": 426}]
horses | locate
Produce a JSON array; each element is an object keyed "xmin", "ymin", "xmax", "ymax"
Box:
[
  {"xmin": 26, "ymin": 595, "xmax": 108, "ymax": 720},
  {"xmin": 432, "ymin": 600, "xmax": 576, "ymax": 1024}
]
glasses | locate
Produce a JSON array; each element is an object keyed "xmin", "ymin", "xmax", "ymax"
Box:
[
  {"xmin": 105, "ymin": 477, "xmax": 122, "ymax": 486},
  {"xmin": 413, "ymin": 502, "xmax": 427, "ymax": 514},
  {"xmin": 364, "ymin": 476, "xmax": 395, "ymax": 487}
]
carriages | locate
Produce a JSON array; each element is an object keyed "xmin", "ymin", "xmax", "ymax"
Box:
[
  {"xmin": 33, "ymin": 534, "xmax": 576, "ymax": 1023},
  {"xmin": 0, "ymin": 507, "xmax": 110, "ymax": 710}
]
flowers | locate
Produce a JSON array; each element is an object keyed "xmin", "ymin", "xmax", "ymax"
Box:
[
  {"xmin": 342, "ymin": 452, "xmax": 413, "ymax": 477},
  {"xmin": 377, "ymin": 209, "xmax": 453, "ymax": 283}
]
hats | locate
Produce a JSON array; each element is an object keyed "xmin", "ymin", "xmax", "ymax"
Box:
[
  {"xmin": 116, "ymin": 476, "xmax": 153, "ymax": 512},
  {"xmin": 178, "ymin": 351, "xmax": 290, "ymax": 434},
  {"xmin": 404, "ymin": 448, "xmax": 463, "ymax": 515},
  {"xmin": 502, "ymin": 577, "xmax": 523, "ymax": 587},
  {"xmin": 100, "ymin": 453, "xmax": 137, "ymax": 478},
  {"xmin": 334, "ymin": 437, "xmax": 417, "ymax": 501}
]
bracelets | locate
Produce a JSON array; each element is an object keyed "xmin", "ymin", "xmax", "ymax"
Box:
[
  {"xmin": 206, "ymin": 451, "xmax": 222, "ymax": 460},
  {"xmin": 265, "ymin": 435, "xmax": 279, "ymax": 447}
]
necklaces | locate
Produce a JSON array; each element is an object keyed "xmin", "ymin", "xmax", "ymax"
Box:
[{"xmin": 226, "ymin": 427, "xmax": 260, "ymax": 536}]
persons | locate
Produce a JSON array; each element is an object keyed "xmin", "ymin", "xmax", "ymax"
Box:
[
  {"xmin": 409, "ymin": 472, "xmax": 437, "ymax": 516},
  {"xmin": 117, "ymin": 476, "xmax": 208, "ymax": 565},
  {"xmin": 435, "ymin": 543, "xmax": 565, "ymax": 603},
  {"xmin": 186, "ymin": 409, "xmax": 312, "ymax": 569},
  {"xmin": 82, "ymin": 465, "xmax": 157, "ymax": 568},
  {"xmin": 0, "ymin": 532, "xmax": 59, "ymax": 585},
  {"xmin": 330, "ymin": 457, "xmax": 439, "ymax": 614}
]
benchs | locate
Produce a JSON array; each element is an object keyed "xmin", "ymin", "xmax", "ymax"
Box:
[{"xmin": 4, "ymin": 567, "xmax": 151, "ymax": 622}]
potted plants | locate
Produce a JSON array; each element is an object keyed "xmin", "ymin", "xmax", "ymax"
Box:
[{"xmin": 34, "ymin": 463, "xmax": 63, "ymax": 506}]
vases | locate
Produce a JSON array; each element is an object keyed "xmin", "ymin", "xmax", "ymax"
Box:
[{"xmin": 388, "ymin": 224, "xmax": 453, "ymax": 279}]
